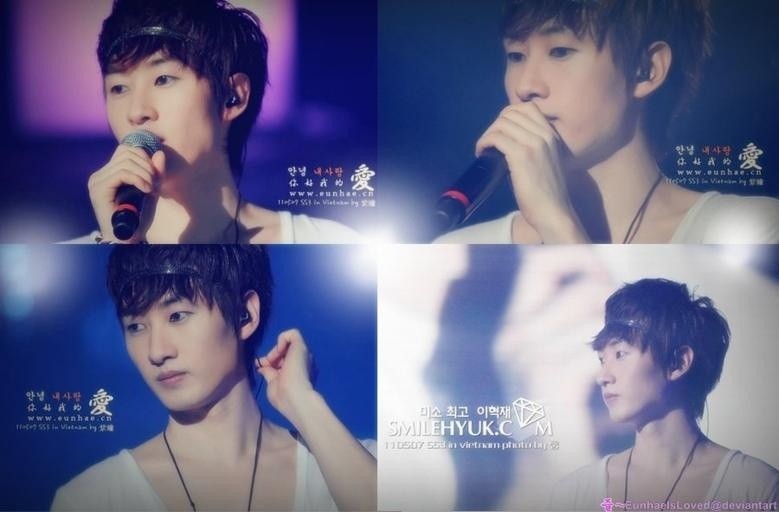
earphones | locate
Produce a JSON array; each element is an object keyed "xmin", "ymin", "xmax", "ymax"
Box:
[
  {"xmin": 672, "ymin": 348, "xmax": 681, "ymax": 370},
  {"xmin": 225, "ymin": 93, "xmax": 238, "ymax": 107},
  {"xmin": 238, "ymin": 308, "xmax": 250, "ymax": 325},
  {"xmin": 638, "ymin": 58, "xmax": 651, "ymax": 82}
]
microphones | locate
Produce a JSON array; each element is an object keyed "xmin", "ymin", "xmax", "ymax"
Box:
[
  {"xmin": 111, "ymin": 132, "xmax": 162, "ymax": 241},
  {"xmin": 431, "ymin": 145, "xmax": 511, "ymax": 231}
]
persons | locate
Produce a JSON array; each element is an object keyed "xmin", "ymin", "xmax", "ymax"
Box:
[
  {"xmin": 49, "ymin": 0, "xmax": 375, "ymax": 245},
  {"xmin": 428, "ymin": 0, "xmax": 779, "ymax": 244},
  {"xmin": 47, "ymin": 245, "xmax": 377, "ymax": 512},
  {"xmin": 547, "ymin": 276, "xmax": 779, "ymax": 512}
]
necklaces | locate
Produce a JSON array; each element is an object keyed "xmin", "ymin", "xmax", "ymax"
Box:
[
  {"xmin": 163, "ymin": 408, "xmax": 264, "ymax": 512},
  {"xmin": 622, "ymin": 173, "xmax": 663, "ymax": 244},
  {"xmin": 232, "ymin": 190, "xmax": 242, "ymax": 244},
  {"xmin": 623, "ymin": 431, "xmax": 704, "ymax": 512}
]
